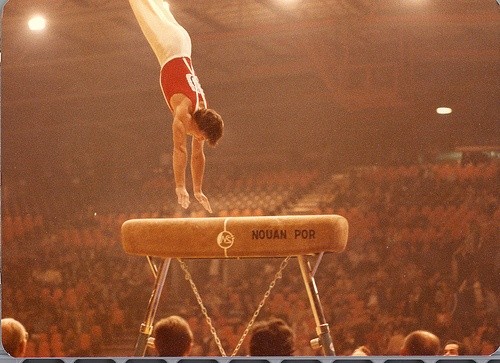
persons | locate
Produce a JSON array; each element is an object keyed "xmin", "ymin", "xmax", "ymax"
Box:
[
  {"xmin": 127, "ymin": 0, "xmax": 224, "ymax": 214},
  {"xmin": 400, "ymin": 330, "xmax": 440, "ymax": 356},
  {"xmin": 0, "ymin": 152, "xmax": 500, "ymax": 363},
  {"xmin": 248, "ymin": 318, "xmax": 296, "ymax": 356},
  {"xmin": 152, "ymin": 315, "xmax": 194, "ymax": 357},
  {"xmin": 443, "ymin": 341, "xmax": 464, "ymax": 356},
  {"xmin": 1, "ymin": 317, "xmax": 29, "ymax": 358}
]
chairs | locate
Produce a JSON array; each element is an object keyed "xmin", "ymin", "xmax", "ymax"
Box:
[{"xmin": 1, "ymin": 165, "xmax": 500, "ymax": 358}]
